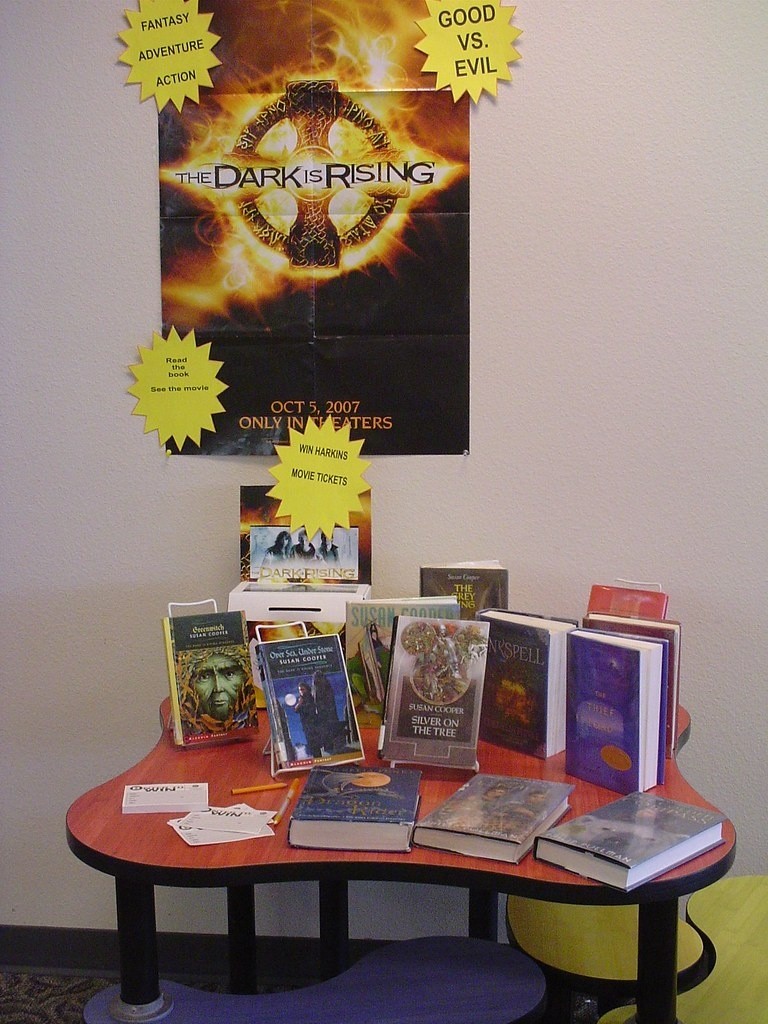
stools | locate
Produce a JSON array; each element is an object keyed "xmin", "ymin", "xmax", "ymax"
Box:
[
  {"xmin": 501, "ymin": 894, "xmax": 703, "ymax": 1024},
  {"xmin": 596, "ymin": 876, "xmax": 768, "ymax": 1024}
]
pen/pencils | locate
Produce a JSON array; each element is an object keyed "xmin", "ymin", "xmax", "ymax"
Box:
[
  {"xmin": 231, "ymin": 782, "xmax": 288, "ymax": 794},
  {"xmin": 274, "ymin": 778, "xmax": 300, "ymax": 826}
]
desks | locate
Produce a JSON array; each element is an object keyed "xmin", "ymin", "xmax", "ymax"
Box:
[{"xmin": 65, "ymin": 694, "xmax": 736, "ymax": 1024}]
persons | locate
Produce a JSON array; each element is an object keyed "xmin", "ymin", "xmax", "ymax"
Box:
[
  {"xmin": 318, "ymin": 531, "xmax": 339, "ymax": 564},
  {"xmin": 266, "ymin": 530, "xmax": 292, "ymax": 559},
  {"xmin": 291, "ymin": 530, "xmax": 323, "ymax": 562},
  {"xmin": 295, "ymin": 670, "xmax": 340, "ymax": 759}
]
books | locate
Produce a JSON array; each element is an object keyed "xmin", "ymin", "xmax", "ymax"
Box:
[
  {"xmin": 164, "ymin": 611, "xmax": 259, "ymax": 746},
  {"xmin": 533, "ymin": 791, "xmax": 727, "ymax": 893},
  {"xmin": 255, "ymin": 634, "xmax": 365, "ymax": 770},
  {"xmin": 564, "ymin": 628, "xmax": 669, "ymax": 793},
  {"xmin": 587, "ymin": 584, "xmax": 668, "ymax": 619},
  {"xmin": 411, "ymin": 773, "xmax": 575, "ymax": 865},
  {"xmin": 376, "ymin": 615, "xmax": 490, "ymax": 770},
  {"xmin": 287, "ymin": 765, "xmax": 423, "ymax": 854},
  {"xmin": 345, "ymin": 596, "xmax": 461, "ymax": 729},
  {"xmin": 476, "ymin": 607, "xmax": 578, "ymax": 760},
  {"xmin": 419, "ymin": 560, "xmax": 508, "ymax": 621},
  {"xmin": 583, "ymin": 610, "xmax": 681, "ymax": 759}
]
